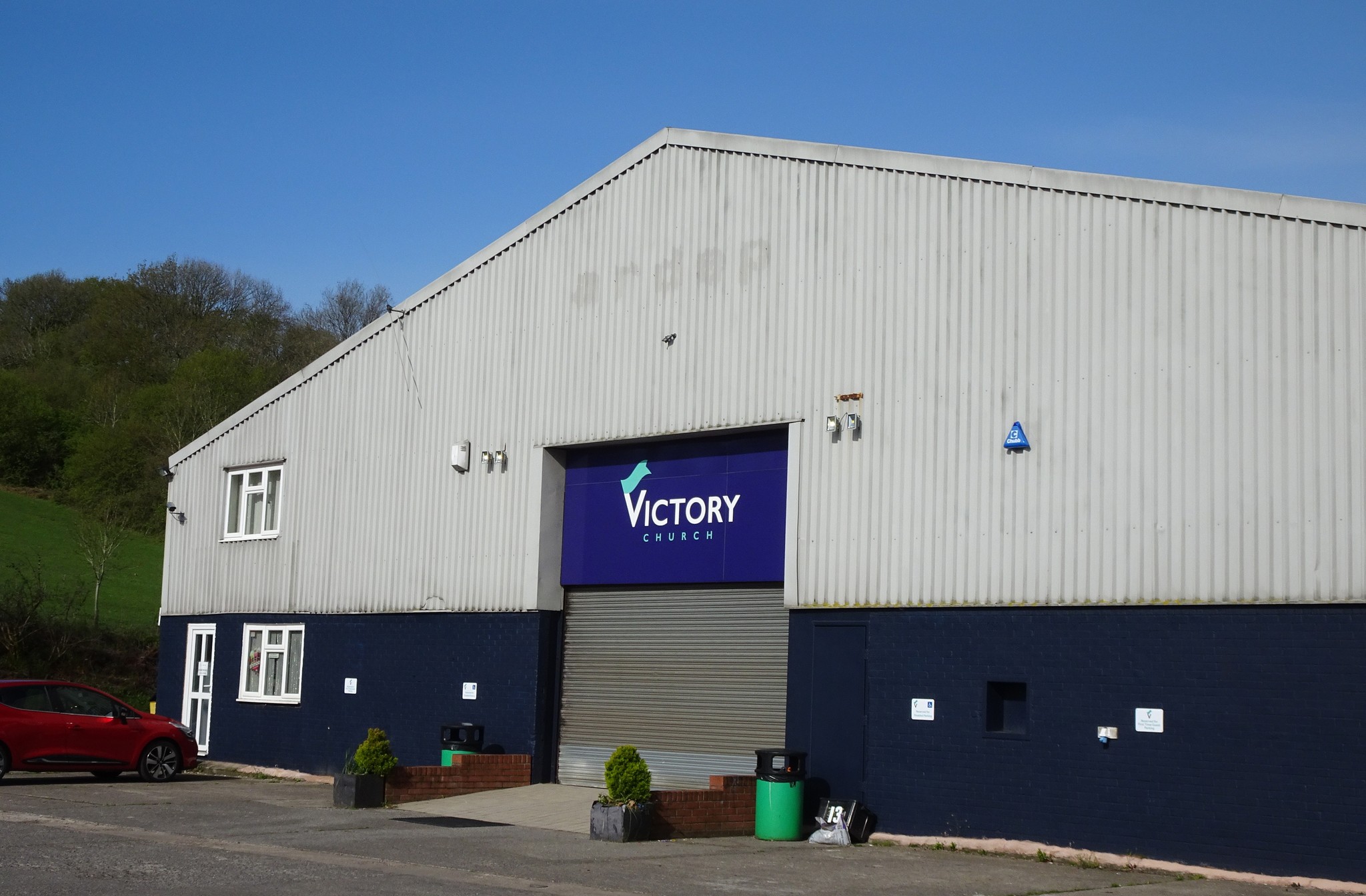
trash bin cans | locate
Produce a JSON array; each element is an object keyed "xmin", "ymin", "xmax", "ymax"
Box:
[
  {"xmin": 440, "ymin": 723, "xmax": 485, "ymax": 766},
  {"xmin": 753, "ymin": 748, "xmax": 807, "ymax": 842}
]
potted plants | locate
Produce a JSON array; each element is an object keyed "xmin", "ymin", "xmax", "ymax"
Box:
[
  {"xmin": 590, "ymin": 745, "xmax": 657, "ymax": 843},
  {"xmin": 334, "ymin": 727, "xmax": 399, "ymax": 809}
]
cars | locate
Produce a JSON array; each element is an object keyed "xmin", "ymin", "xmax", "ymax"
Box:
[{"xmin": 0, "ymin": 677, "xmax": 204, "ymax": 783}]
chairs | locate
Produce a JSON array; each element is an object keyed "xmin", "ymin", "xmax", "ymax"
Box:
[{"xmin": 53, "ymin": 694, "xmax": 66, "ymax": 713}]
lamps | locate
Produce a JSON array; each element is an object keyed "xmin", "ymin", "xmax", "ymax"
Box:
[
  {"xmin": 826, "ymin": 415, "xmax": 840, "ymax": 432},
  {"xmin": 662, "ymin": 333, "xmax": 677, "ymax": 344},
  {"xmin": 846, "ymin": 412, "xmax": 861, "ymax": 430},
  {"xmin": 494, "ymin": 451, "xmax": 506, "ymax": 464},
  {"xmin": 157, "ymin": 466, "xmax": 173, "ymax": 478},
  {"xmin": 481, "ymin": 450, "xmax": 493, "ymax": 464}
]
